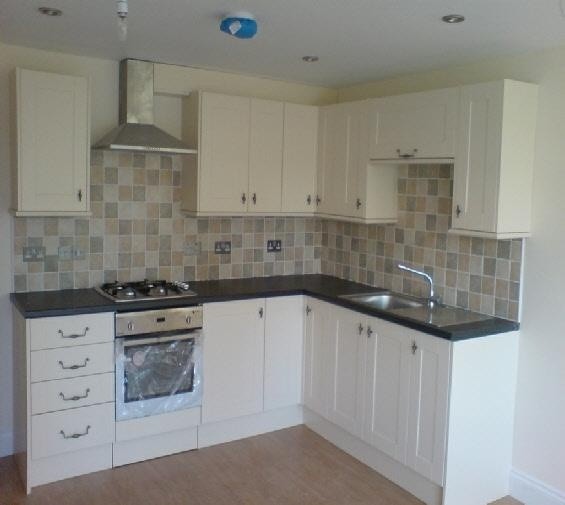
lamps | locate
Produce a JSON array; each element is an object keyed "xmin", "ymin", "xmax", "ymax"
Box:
[{"xmin": 117, "ymin": 1, "xmax": 130, "ymax": 40}]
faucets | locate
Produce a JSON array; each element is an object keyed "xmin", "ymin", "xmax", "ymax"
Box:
[{"xmin": 395, "ymin": 262, "xmax": 441, "ymax": 308}]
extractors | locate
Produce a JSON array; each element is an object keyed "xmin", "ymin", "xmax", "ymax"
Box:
[{"xmin": 90, "ymin": 58, "xmax": 198, "ymax": 159}]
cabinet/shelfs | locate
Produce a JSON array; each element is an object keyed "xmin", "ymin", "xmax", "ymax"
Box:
[
  {"xmin": 335, "ymin": 304, "xmax": 408, "ymax": 493},
  {"xmin": 201, "ymin": 297, "xmax": 264, "ymax": 449},
  {"xmin": 368, "ymin": 84, "xmax": 459, "ymax": 165},
  {"xmin": 264, "ymin": 295, "xmax": 303, "ymax": 435},
  {"xmin": 407, "ymin": 328, "xmax": 520, "ymax": 505},
  {"xmin": 8, "ymin": 66, "xmax": 92, "ymax": 217},
  {"xmin": 12, "ymin": 304, "xmax": 116, "ymax": 496},
  {"xmin": 303, "ymin": 294, "xmax": 335, "ymax": 443},
  {"xmin": 179, "ymin": 88, "xmax": 285, "ymax": 215},
  {"xmin": 448, "ymin": 78, "xmax": 540, "ymax": 240},
  {"xmin": 347, "ymin": 98, "xmax": 399, "ymax": 225},
  {"xmin": 282, "ymin": 103, "xmax": 319, "ymax": 218},
  {"xmin": 319, "ymin": 102, "xmax": 347, "ymax": 222}
]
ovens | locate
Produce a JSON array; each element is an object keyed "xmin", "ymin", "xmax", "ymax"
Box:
[{"xmin": 115, "ymin": 337, "xmax": 204, "ymax": 422}]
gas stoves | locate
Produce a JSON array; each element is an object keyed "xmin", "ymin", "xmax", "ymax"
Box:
[{"xmin": 94, "ymin": 277, "xmax": 205, "ymax": 337}]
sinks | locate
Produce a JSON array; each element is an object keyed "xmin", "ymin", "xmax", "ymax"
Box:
[{"xmin": 336, "ymin": 286, "xmax": 425, "ymax": 311}]
